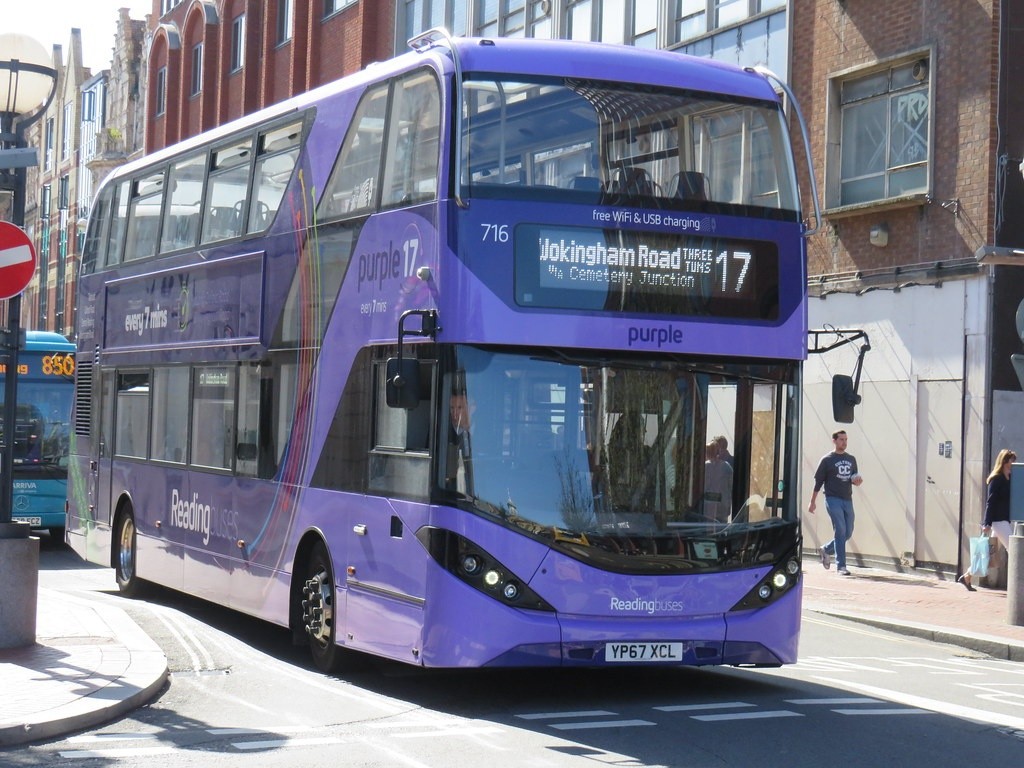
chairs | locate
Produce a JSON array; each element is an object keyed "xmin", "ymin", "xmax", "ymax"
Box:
[
  {"xmin": 401, "ymin": 191, "xmax": 435, "ymax": 202},
  {"xmin": 568, "ymin": 171, "xmax": 712, "ymax": 203},
  {"xmin": 109, "ymin": 200, "xmax": 344, "ymax": 267}
]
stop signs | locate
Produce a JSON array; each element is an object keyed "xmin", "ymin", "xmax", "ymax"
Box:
[{"xmin": 0, "ymin": 221, "xmax": 39, "ymax": 301}]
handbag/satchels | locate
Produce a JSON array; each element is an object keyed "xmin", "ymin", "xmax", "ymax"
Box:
[{"xmin": 969, "ymin": 532, "xmax": 990, "ymax": 577}]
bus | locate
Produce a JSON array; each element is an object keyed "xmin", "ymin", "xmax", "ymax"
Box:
[
  {"xmin": 63, "ymin": 26, "xmax": 873, "ymax": 672},
  {"xmin": 0, "ymin": 325, "xmax": 74, "ymax": 544}
]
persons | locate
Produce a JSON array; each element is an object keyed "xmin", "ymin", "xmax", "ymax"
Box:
[
  {"xmin": 808, "ymin": 430, "xmax": 863, "ymax": 575},
  {"xmin": 957, "ymin": 449, "xmax": 1016, "ymax": 591},
  {"xmin": 426, "ymin": 386, "xmax": 503, "ymax": 479},
  {"xmin": 703, "ymin": 436, "xmax": 734, "ymax": 523},
  {"xmin": 596, "ymin": 413, "xmax": 655, "ymax": 513}
]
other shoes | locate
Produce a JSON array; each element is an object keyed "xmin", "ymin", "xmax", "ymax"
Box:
[
  {"xmin": 957, "ymin": 575, "xmax": 977, "ymax": 592},
  {"xmin": 820, "ymin": 545, "xmax": 831, "ymax": 569},
  {"xmin": 837, "ymin": 566, "xmax": 851, "ymax": 575}
]
